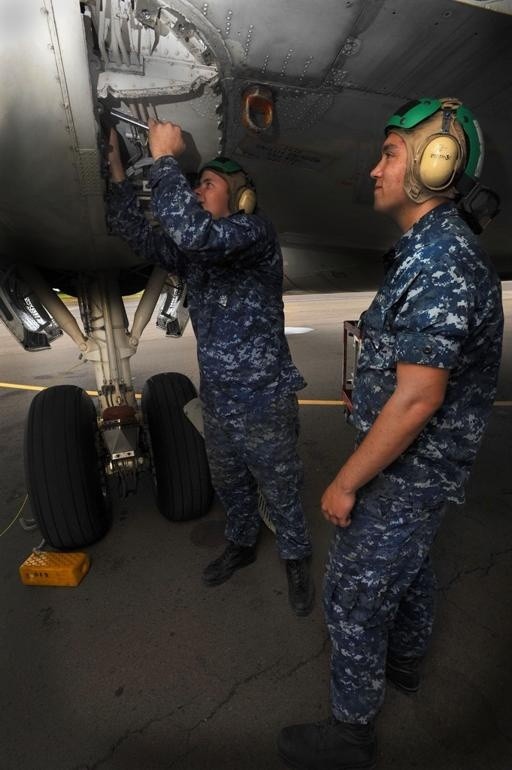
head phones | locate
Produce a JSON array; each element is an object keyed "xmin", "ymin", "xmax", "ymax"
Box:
[
  {"xmin": 228, "ymin": 174, "xmax": 259, "ymax": 216},
  {"xmin": 415, "ymin": 99, "xmax": 465, "ymax": 193}
]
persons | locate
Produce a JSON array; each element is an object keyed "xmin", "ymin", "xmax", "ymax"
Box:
[
  {"xmin": 102, "ymin": 117, "xmax": 315, "ymax": 616},
  {"xmin": 278, "ymin": 98, "xmax": 506, "ymax": 763}
]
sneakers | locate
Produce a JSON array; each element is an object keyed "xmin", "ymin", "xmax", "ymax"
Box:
[
  {"xmin": 385, "ymin": 654, "xmax": 422, "ymax": 693},
  {"xmin": 201, "ymin": 543, "xmax": 258, "ymax": 587},
  {"xmin": 276, "ymin": 716, "xmax": 376, "ymax": 770},
  {"xmin": 286, "ymin": 558, "xmax": 314, "ymax": 618}
]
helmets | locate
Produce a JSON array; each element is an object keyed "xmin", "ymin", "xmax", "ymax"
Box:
[
  {"xmin": 384, "ymin": 95, "xmax": 486, "ymax": 204},
  {"xmin": 198, "ymin": 156, "xmax": 256, "ymax": 214}
]
